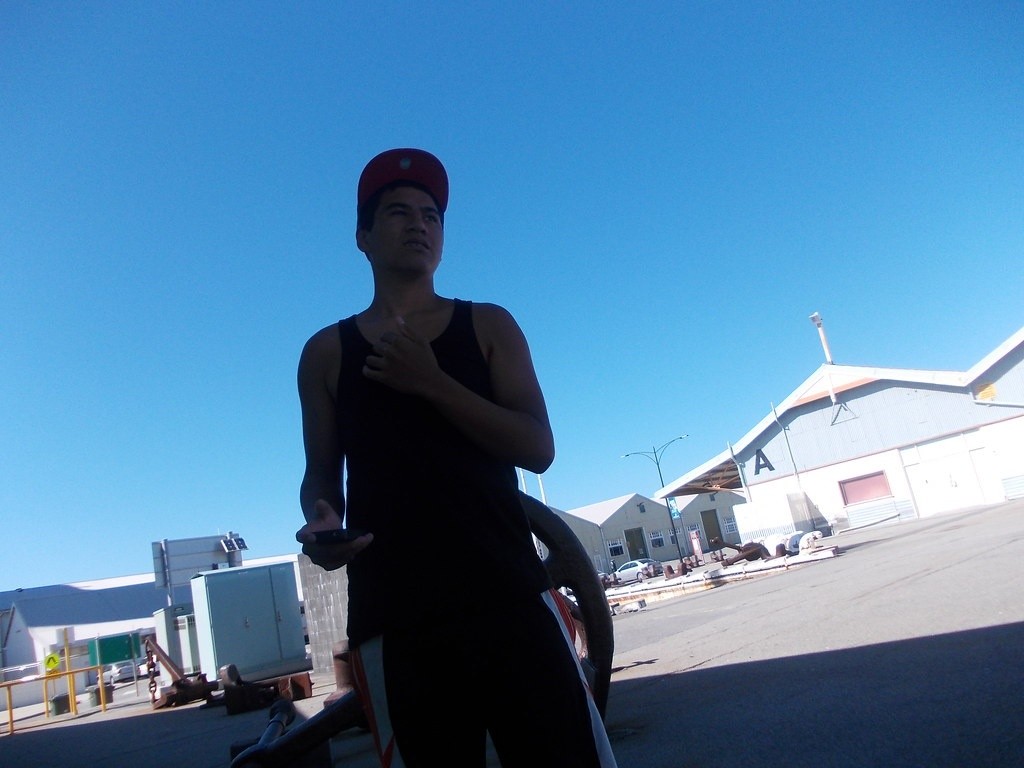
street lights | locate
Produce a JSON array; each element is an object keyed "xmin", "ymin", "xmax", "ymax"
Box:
[{"xmin": 620, "ymin": 435, "xmax": 688, "ymax": 566}]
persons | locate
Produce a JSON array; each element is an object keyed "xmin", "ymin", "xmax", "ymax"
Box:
[{"xmin": 295, "ymin": 148, "xmax": 622, "ymax": 768}]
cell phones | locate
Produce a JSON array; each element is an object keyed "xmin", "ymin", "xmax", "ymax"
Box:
[{"xmin": 313, "ymin": 529, "xmax": 360, "ymax": 545}]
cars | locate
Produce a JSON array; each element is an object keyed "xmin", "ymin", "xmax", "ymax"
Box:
[
  {"xmin": 614, "ymin": 557, "xmax": 663, "ymax": 582},
  {"xmin": 596, "ymin": 569, "xmax": 611, "ymax": 590},
  {"xmin": 96, "ymin": 659, "xmax": 136, "ymax": 686},
  {"xmin": 136, "ymin": 654, "xmax": 160, "ymax": 677}
]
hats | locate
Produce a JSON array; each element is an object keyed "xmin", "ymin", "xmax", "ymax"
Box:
[{"xmin": 357, "ymin": 147, "xmax": 449, "ymax": 239}]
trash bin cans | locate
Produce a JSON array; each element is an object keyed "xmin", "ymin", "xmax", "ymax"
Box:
[
  {"xmin": 85, "ymin": 684, "xmax": 116, "ymax": 709},
  {"xmin": 49, "ymin": 692, "xmax": 71, "ymax": 716}
]
